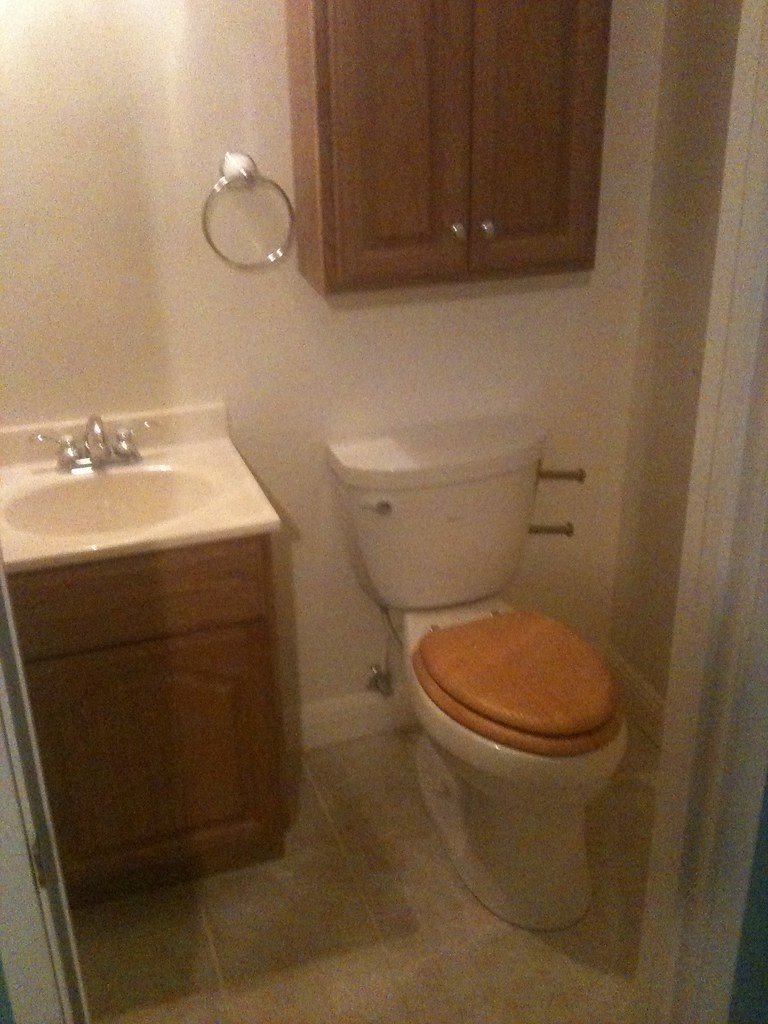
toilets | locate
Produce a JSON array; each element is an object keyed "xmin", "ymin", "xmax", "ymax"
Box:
[{"xmin": 323, "ymin": 414, "xmax": 631, "ymax": 933}]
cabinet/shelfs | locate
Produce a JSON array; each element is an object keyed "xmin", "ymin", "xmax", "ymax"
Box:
[
  {"xmin": 285, "ymin": 0, "xmax": 616, "ymax": 298},
  {"xmin": 4, "ymin": 532, "xmax": 302, "ymax": 911}
]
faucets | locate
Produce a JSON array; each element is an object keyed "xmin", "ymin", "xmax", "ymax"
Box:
[{"xmin": 32, "ymin": 413, "xmax": 152, "ymax": 470}]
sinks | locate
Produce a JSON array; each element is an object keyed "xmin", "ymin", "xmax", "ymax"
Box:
[{"xmin": 1, "ymin": 467, "xmax": 214, "ymax": 540}]
